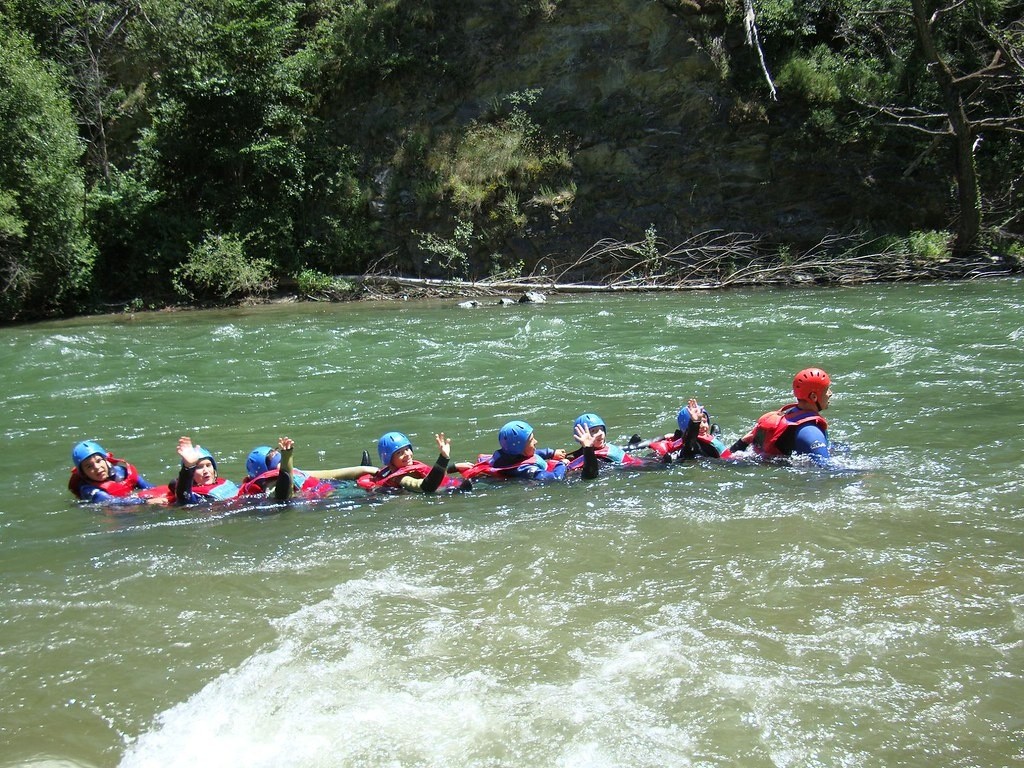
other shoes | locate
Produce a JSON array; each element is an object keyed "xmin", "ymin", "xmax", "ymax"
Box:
[
  {"xmin": 361, "ymin": 450, "xmax": 372, "ymax": 466},
  {"xmin": 710, "ymin": 423, "xmax": 721, "ymax": 440},
  {"xmin": 628, "ymin": 434, "xmax": 641, "ymax": 445}
]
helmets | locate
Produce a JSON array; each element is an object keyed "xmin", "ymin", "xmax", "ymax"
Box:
[
  {"xmin": 793, "ymin": 368, "xmax": 830, "ymax": 404},
  {"xmin": 245, "ymin": 447, "xmax": 274, "ymax": 478},
  {"xmin": 378, "ymin": 431, "xmax": 413, "ymax": 465},
  {"xmin": 573, "ymin": 413, "xmax": 606, "ymax": 442},
  {"xmin": 498, "ymin": 420, "xmax": 534, "ymax": 455},
  {"xmin": 181, "ymin": 446, "xmax": 217, "ymax": 471},
  {"xmin": 677, "ymin": 404, "xmax": 710, "ymax": 433},
  {"xmin": 72, "ymin": 440, "xmax": 107, "ymax": 473}
]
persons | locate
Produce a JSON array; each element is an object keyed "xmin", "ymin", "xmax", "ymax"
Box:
[
  {"xmin": 753, "ymin": 368, "xmax": 833, "ymax": 458},
  {"xmin": 176, "ymin": 437, "xmax": 239, "ymax": 504},
  {"xmin": 677, "ymin": 399, "xmax": 754, "ymax": 459},
  {"xmin": 476, "ymin": 420, "xmax": 570, "ymax": 482},
  {"xmin": 238, "ymin": 436, "xmax": 320, "ymax": 500},
  {"xmin": 374, "ymin": 432, "xmax": 475, "ymax": 492},
  {"xmin": 67, "ymin": 441, "xmax": 168, "ymax": 505},
  {"xmin": 567, "ymin": 413, "xmax": 635, "ymax": 479}
]
